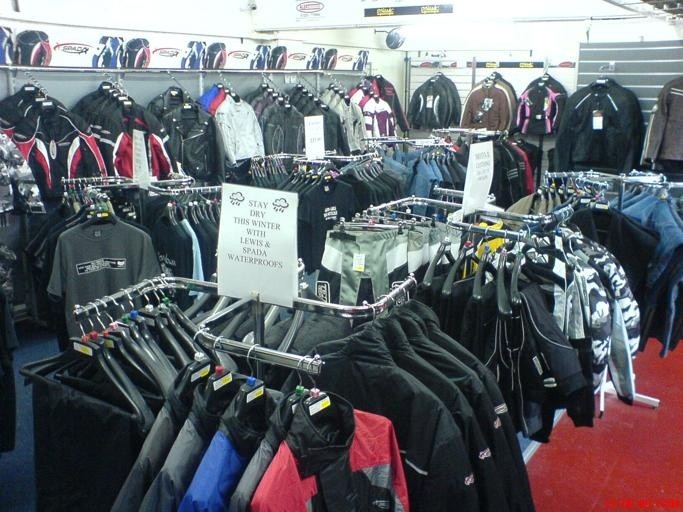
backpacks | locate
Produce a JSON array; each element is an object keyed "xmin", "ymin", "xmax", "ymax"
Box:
[
  {"xmin": 126, "ymin": 39, "xmax": 150, "ymax": 70},
  {"xmin": 271, "ymin": 46, "xmax": 287, "ymax": 70},
  {"xmin": 353, "ymin": 51, "xmax": 369, "ymax": 70},
  {"xmin": 181, "ymin": 42, "xmax": 206, "ymax": 70},
  {"xmin": 307, "ymin": 48, "xmax": 325, "ymax": 70},
  {"xmin": 206, "ymin": 43, "xmax": 226, "ymax": 69},
  {"xmin": 325, "ymin": 50, "xmax": 337, "ymax": 70},
  {"xmin": 92, "ymin": 36, "xmax": 126, "ymax": 67},
  {"xmin": 250, "ymin": 45, "xmax": 270, "ymax": 69},
  {"xmin": 0, "ymin": 26, "xmax": 15, "ymax": 66},
  {"xmin": 14, "ymin": 30, "xmax": 51, "ymax": 66}
]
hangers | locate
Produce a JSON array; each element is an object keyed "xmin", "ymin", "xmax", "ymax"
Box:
[{"xmin": 1, "ymin": 69, "xmax": 683, "ymax": 432}]
